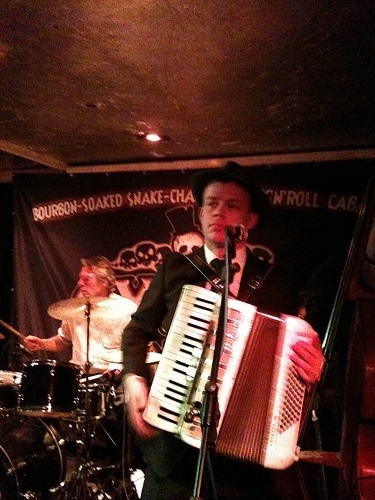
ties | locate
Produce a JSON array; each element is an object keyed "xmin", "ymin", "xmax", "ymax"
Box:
[{"xmin": 211, "ymin": 259, "xmax": 233, "ymax": 295}]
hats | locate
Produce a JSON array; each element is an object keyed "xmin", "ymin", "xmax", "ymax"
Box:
[{"xmin": 193, "ymin": 160, "xmax": 271, "ymax": 234}]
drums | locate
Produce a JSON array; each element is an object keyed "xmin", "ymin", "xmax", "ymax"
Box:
[
  {"xmin": 81, "ymin": 375, "xmax": 114, "ymax": 419},
  {"xmin": 16, "ymin": 359, "xmax": 81, "ymax": 418},
  {"xmin": 0, "ymin": 369, "xmax": 22, "ymax": 405},
  {"xmin": 0, "ymin": 418, "xmax": 67, "ymax": 500}
]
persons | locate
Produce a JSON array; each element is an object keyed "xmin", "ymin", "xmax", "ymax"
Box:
[
  {"xmin": 121, "ymin": 160, "xmax": 325, "ymax": 500},
  {"xmin": 23, "ymin": 256, "xmax": 139, "ymax": 373}
]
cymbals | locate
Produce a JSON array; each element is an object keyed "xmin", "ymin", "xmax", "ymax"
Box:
[{"xmin": 47, "ymin": 296, "xmax": 134, "ymax": 325}]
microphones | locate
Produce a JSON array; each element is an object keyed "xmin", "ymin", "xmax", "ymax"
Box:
[{"xmin": 226, "ymin": 223, "xmax": 248, "ymax": 243}]
